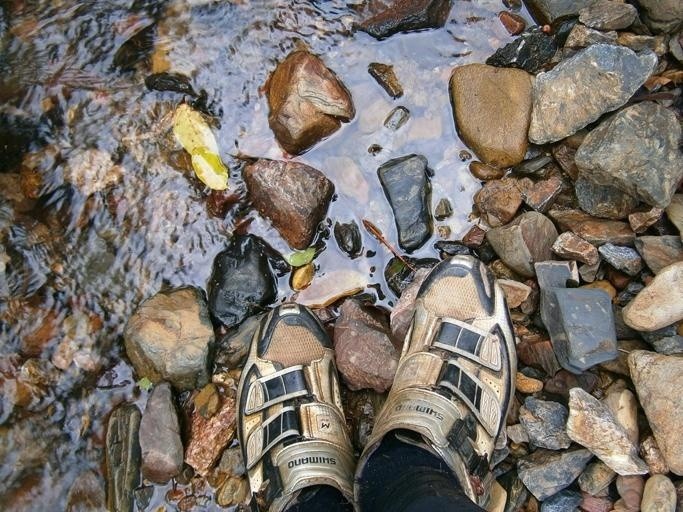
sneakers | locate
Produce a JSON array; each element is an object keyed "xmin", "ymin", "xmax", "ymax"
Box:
[
  {"xmin": 354, "ymin": 253, "xmax": 517, "ymax": 512},
  {"xmin": 235, "ymin": 300, "xmax": 356, "ymax": 512}
]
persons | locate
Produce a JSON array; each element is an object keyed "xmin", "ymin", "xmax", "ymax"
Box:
[{"xmin": 233, "ymin": 254, "xmax": 519, "ymax": 512}]
